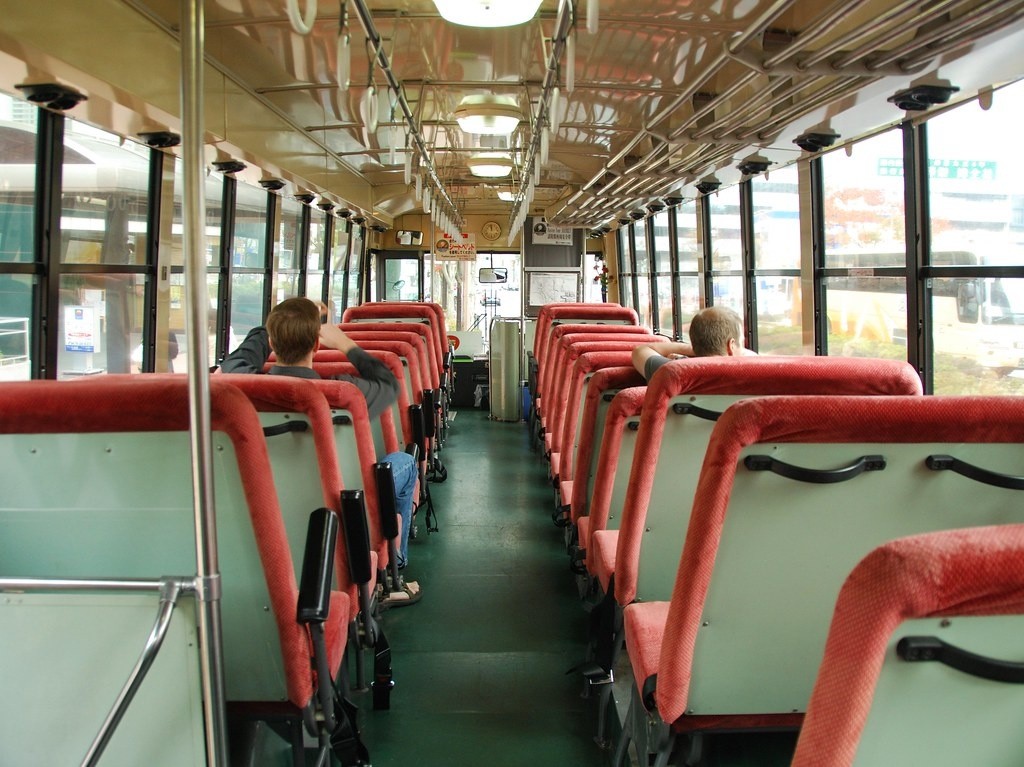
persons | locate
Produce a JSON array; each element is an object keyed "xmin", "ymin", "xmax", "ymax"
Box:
[
  {"xmin": 631, "ymin": 306, "xmax": 761, "ymax": 386},
  {"xmin": 212, "ymin": 295, "xmax": 422, "ymax": 614}
]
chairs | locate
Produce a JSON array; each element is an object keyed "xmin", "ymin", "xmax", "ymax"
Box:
[{"xmin": 0, "ymin": 298, "xmax": 1023, "ymax": 766}]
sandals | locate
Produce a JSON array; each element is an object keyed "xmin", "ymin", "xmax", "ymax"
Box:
[{"xmin": 387, "ymin": 581, "xmax": 423, "ymax": 606}]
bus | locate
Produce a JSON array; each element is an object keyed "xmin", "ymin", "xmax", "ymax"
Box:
[{"xmin": 784, "ymin": 245, "xmax": 1022, "ymax": 374}]
